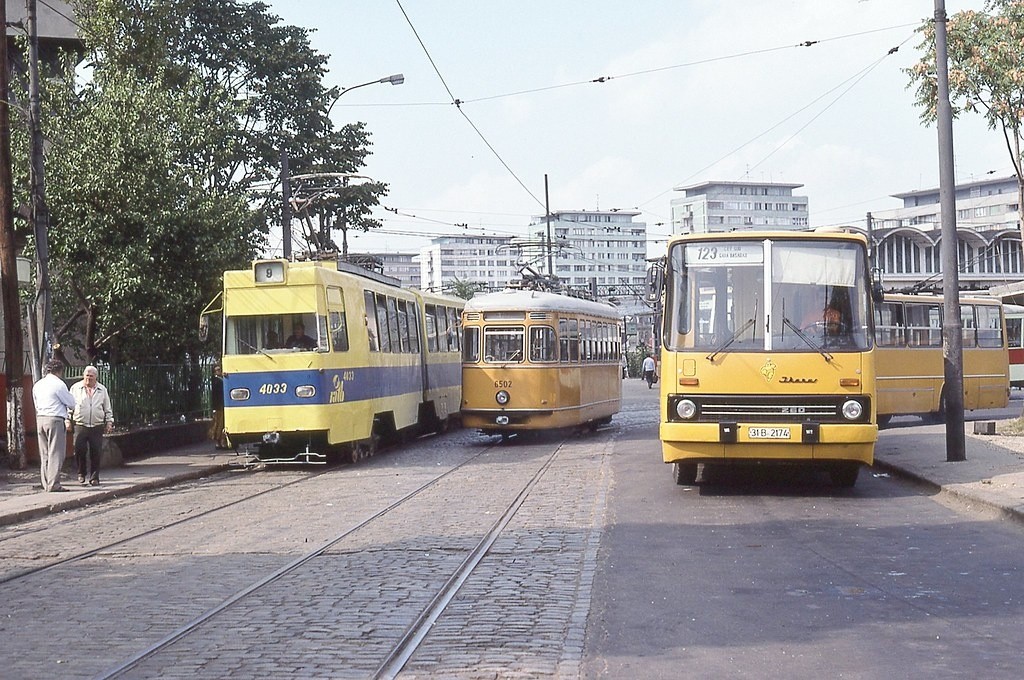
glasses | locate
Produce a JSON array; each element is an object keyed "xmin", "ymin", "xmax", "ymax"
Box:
[{"xmin": 215, "ymin": 369, "xmax": 220, "ymax": 371}]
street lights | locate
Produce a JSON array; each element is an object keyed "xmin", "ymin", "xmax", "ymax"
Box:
[{"xmin": 320, "ymin": 73, "xmax": 405, "ymax": 251}]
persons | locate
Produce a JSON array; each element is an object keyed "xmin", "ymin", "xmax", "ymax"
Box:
[
  {"xmin": 799, "ymin": 286, "xmax": 841, "ymax": 336},
  {"xmin": 64, "ymin": 366, "xmax": 115, "ymax": 484},
  {"xmin": 622, "ymin": 352, "xmax": 627, "ymax": 379},
  {"xmin": 641, "ymin": 352, "xmax": 657, "ymax": 389},
  {"xmin": 285, "ymin": 323, "xmax": 317, "ymax": 349},
  {"xmin": 207, "ymin": 365, "xmax": 226, "ymax": 449},
  {"xmin": 32, "ymin": 359, "xmax": 76, "ymax": 492},
  {"xmin": 266, "ymin": 331, "xmax": 285, "ymax": 350}
]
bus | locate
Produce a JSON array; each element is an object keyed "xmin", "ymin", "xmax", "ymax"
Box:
[
  {"xmin": 200, "ymin": 259, "xmax": 469, "ymax": 467},
  {"xmin": 644, "ymin": 227, "xmax": 879, "ymax": 485},
  {"xmin": 458, "ymin": 289, "xmax": 622, "ymax": 437},
  {"xmin": 871, "ymin": 294, "xmax": 1010, "ymax": 424},
  {"xmin": 874, "ymin": 304, "xmax": 1024, "ymax": 386}
]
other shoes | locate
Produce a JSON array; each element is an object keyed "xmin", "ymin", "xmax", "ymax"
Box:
[
  {"xmin": 215, "ymin": 444, "xmax": 227, "ymax": 450},
  {"xmin": 50, "ymin": 486, "xmax": 70, "ymax": 492},
  {"xmin": 77, "ymin": 477, "xmax": 85, "ymax": 483},
  {"xmin": 91, "ymin": 480, "xmax": 99, "ymax": 486}
]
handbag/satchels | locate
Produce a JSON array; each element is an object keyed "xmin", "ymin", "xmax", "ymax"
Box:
[{"xmin": 652, "ymin": 374, "xmax": 659, "ymax": 383}]
